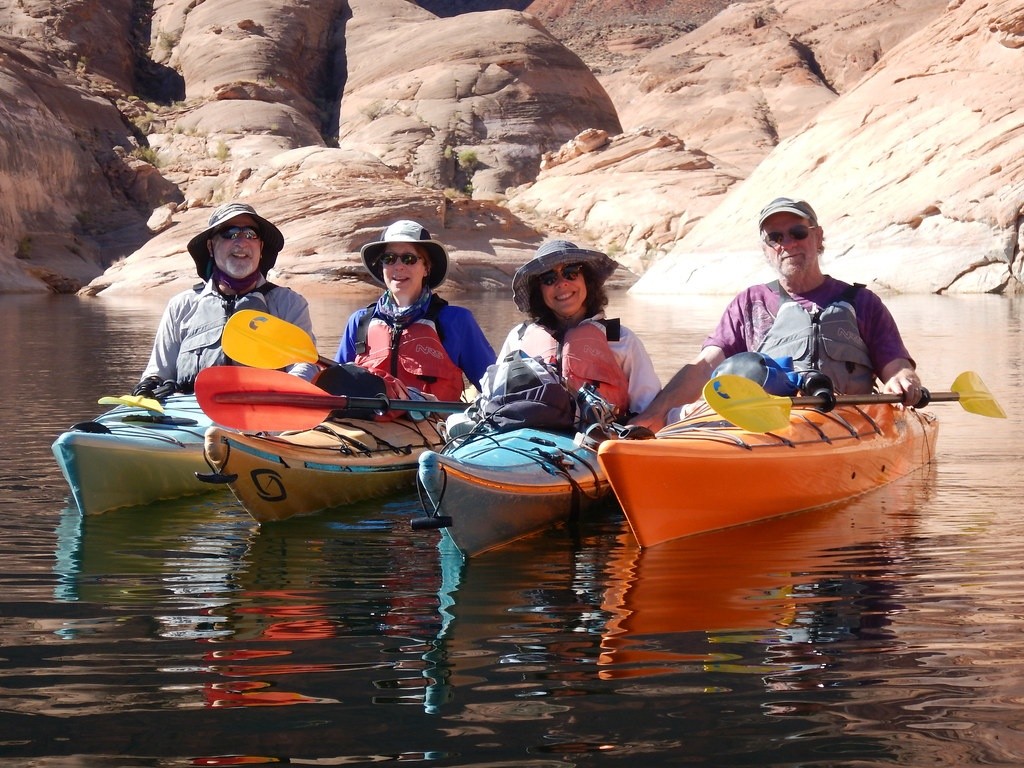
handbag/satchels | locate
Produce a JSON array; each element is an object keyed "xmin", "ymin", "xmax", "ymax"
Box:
[{"xmin": 477, "ymin": 353, "xmax": 582, "ymax": 435}]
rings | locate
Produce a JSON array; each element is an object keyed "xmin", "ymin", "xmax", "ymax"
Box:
[{"xmin": 914, "ymin": 388, "xmax": 919, "ymax": 391}]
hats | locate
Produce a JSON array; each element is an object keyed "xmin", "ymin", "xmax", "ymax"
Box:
[
  {"xmin": 511, "ymin": 240, "xmax": 619, "ymax": 317},
  {"xmin": 187, "ymin": 203, "xmax": 284, "ymax": 282},
  {"xmin": 360, "ymin": 220, "xmax": 450, "ymax": 289},
  {"xmin": 758, "ymin": 198, "xmax": 817, "ymax": 232}
]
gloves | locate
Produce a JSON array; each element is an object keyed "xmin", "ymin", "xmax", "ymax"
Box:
[{"xmin": 130, "ymin": 378, "xmax": 162, "ymax": 397}]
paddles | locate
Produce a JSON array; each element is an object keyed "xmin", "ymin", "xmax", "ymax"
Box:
[
  {"xmin": 195, "ymin": 365, "xmax": 473, "ymax": 433},
  {"xmin": 219, "ymin": 307, "xmax": 338, "ymax": 370},
  {"xmin": 97, "ymin": 379, "xmax": 177, "ymax": 411},
  {"xmin": 704, "ymin": 370, "xmax": 1009, "ymax": 434}
]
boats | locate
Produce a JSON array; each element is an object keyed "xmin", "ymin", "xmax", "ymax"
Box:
[
  {"xmin": 51, "ymin": 394, "xmax": 938, "ymax": 559},
  {"xmin": 596, "ymin": 460, "xmax": 948, "ymax": 673}
]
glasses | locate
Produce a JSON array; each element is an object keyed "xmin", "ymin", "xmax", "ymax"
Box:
[
  {"xmin": 534, "ymin": 261, "xmax": 584, "ymax": 286},
  {"xmin": 379, "ymin": 252, "xmax": 422, "ymax": 266},
  {"xmin": 762, "ymin": 224, "xmax": 818, "ymax": 248},
  {"xmin": 209, "ymin": 225, "xmax": 262, "ymax": 240}
]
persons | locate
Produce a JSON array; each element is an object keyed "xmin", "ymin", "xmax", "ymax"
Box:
[
  {"xmin": 334, "ymin": 220, "xmax": 498, "ymax": 417},
  {"xmin": 131, "ymin": 204, "xmax": 319, "ymax": 399},
  {"xmin": 446, "ymin": 241, "xmax": 661, "ymax": 439},
  {"xmin": 629, "ymin": 197, "xmax": 922, "ymax": 435}
]
